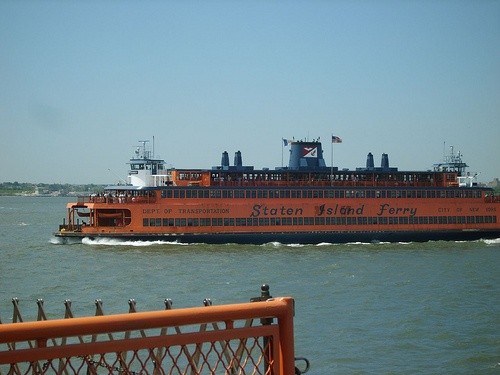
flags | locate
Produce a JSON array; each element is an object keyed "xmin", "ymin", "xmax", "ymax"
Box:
[{"xmin": 332, "ymin": 136, "xmax": 343, "ymax": 144}]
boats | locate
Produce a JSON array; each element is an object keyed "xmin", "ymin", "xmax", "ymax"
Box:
[{"xmin": 53, "ymin": 133, "xmax": 500, "ymax": 245}]
